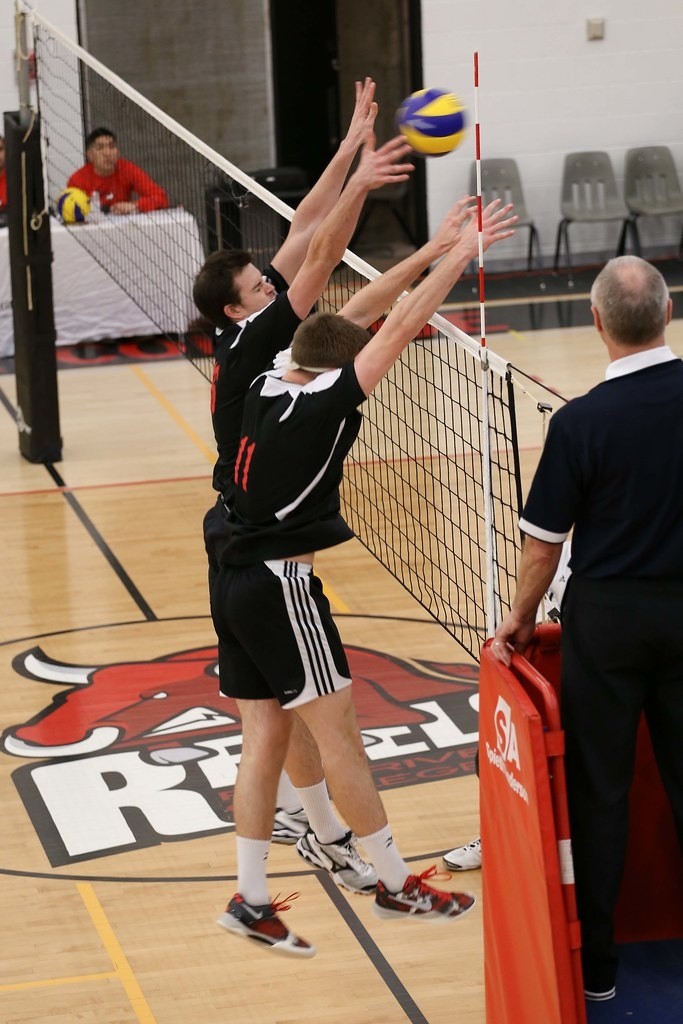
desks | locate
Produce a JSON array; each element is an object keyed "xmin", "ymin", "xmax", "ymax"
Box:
[{"xmin": 0, "ymin": 207, "xmax": 209, "ymax": 359}]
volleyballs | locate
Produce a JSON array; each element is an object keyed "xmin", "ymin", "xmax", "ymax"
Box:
[
  {"xmin": 397, "ymin": 89, "xmax": 469, "ymax": 157},
  {"xmin": 58, "ymin": 187, "xmax": 91, "ymax": 223}
]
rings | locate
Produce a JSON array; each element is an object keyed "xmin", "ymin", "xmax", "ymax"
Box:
[{"xmin": 493, "ymin": 641, "xmax": 499, "ymax": 646}]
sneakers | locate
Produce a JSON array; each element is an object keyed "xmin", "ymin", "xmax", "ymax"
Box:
[
  {"xmin": 583, "ymin": 965, "xmax": 619, "ymax": 1002},
  {"xmin": 272, "ymin": 802, "xmax": 381, "ymax": 894},
  {"xmin": 376, "ymin": 865, "xmax": 476, "ymax": 924},
  {"xmin": 220, "ymin": 891, "xmax": 320, "ymax": 959},
  {"xmin": 445, "ymin": 832, "xmax": 485, "ymax": 872},
  {"xmin": 100, "ymin": 339, "xmax": 166, "ymax": 354}
]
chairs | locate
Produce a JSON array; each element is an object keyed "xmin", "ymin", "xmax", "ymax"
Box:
[
  {"xmin": 552, "ymin": 150, "xmax": 643, "ymax": 289},
  {"xmin": 618, "ymin": 145, "xmax": 683, "ymax": 266},
  {"xmin": 468, "ymin": 159, "xmax": 547, "ymax": 286}
]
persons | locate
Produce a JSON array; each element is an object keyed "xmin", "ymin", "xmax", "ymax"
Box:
[
  {"xmin": 67, "ymin": 127, "xmax": 168, "ymax": 215},
  {"xmin": 0, "ymin": 136, "xmax": 8, "ymax": 214},
  {"xmin": 193, "ymin": 76, "xmax": 416, "ymax": 896},
  {"xmin": 200, "ymin": 196, "xmax": 519, "ymax": 959},
  {"xmin": 490, "ymin": 248, "xmax": 683, "ymax": 1024}
]
